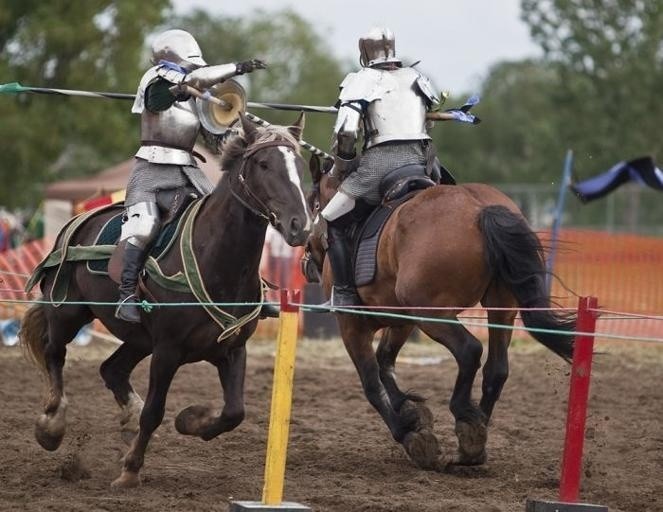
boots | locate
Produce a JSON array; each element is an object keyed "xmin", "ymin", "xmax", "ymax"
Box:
[
  {"xmin": 116, "ymin": 248, "xmax": 141, "ymax": 323},
  {"xmin": 316, "ymin": 222, "xmax": 360, "ymax": 307}
]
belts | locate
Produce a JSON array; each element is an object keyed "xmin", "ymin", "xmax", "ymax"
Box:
[{"xmin": 140, "ymin": 140, "xmax": 207, "ymax": 164}]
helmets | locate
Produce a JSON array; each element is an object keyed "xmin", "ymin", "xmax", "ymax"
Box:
[
  {"xmin": 149, "ymin": 29, "xmax": 207, "ymax": 73},
  {"xmin": 358, "ymin": 26, "xmax": 401, "ymax": 67}
]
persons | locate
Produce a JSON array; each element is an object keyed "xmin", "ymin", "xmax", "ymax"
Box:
[
  {"xmin": 312, "ymin": 25, "xmax": 456, "ymax": 313},
  {"xmin": 117, "ymin": 29, "xmax": 266, "ymax": 324}
]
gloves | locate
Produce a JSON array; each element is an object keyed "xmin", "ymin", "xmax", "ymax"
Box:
[{"xmin": 235, "ymin": 59, "xmax": 268, "ymax": 75}]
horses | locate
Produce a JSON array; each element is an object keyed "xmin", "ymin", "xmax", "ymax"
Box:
[
  {"xmin": 17, "ymin": 108, "xmax": 317, "ymax": 492},
  {"xmin": 295, "ymin": 146, "xmax": 603, "ymax": 469}
]
